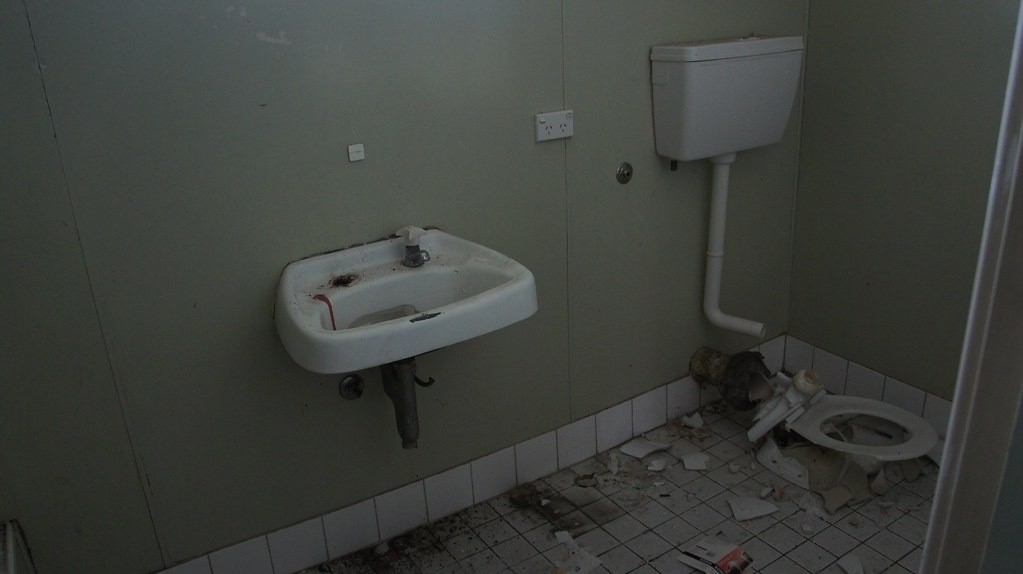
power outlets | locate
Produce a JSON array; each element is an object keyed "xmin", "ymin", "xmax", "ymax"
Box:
[{"xmin": 535, "ymin": 109, "xmax": 575, "ymax": 141}]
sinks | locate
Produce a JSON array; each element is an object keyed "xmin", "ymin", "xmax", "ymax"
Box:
[{"xmin": 273, "ymin": 226, "xmax": 541, "ymax": 377}]
